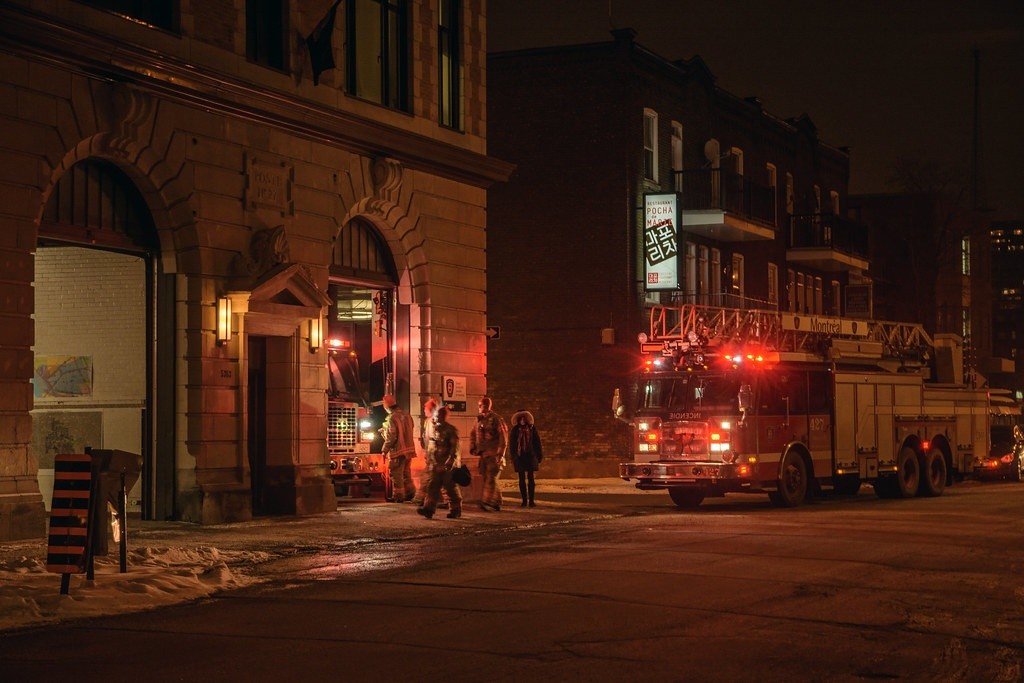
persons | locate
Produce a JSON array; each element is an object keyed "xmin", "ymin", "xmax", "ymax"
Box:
[
  {"xmin": 469, "ymin": 397, "xmax": 508, "ymax": 512},
  {"xmin": 414, "ymin": 399, "xmax": 462, "ymax": 520},
  {"xmin": 509, "ymin": 409, "xmax": 544, "ymax": 507},
  {"xmin": 380, "ymin": 396, "xmax": 417, "ymax": 503}
]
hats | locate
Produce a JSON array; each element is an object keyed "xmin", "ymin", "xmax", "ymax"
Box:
[
  {"xmin": 424, "ymin": 399, "xmax": 438, "ymax": 412},
  {"xmin": 383, "ymin": 395, "xmax": 397, "ymax": 408}
]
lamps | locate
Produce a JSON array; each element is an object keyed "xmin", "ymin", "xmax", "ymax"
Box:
[
  {"xmin": 216, "ymin": 297, "xmax": 233, "ymax": 347},
  {"xmin": 309, "ymin": 311, "xmax": 323, "ymax": 353}
]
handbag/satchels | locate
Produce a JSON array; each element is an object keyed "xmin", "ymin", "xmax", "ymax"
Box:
[
  {"xmin": 532, "ymin": 453, "xmax": 539, "ymax": 471},
  {"xmin": 454, "ymin": 464, "xmax": 471, "ymax": 487}
]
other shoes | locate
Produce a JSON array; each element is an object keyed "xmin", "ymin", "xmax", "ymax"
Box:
[
  {"xmin": 417, "ymin": 506, "xmax": 433, "ymax": 519},
  {"xmin": 386, "ymin": 496, "xmax": 402, "ymax": 503},
  {"xmin": 447, "ymin": 510, "xmax": 462, "ymax": 518},
  {"xmin": 402, "ymin": 496, "xmax": 413, "ymax": 501},
  {"xmin": 478, "ymin": 501, "xmax": 500, "ymax": 512}
]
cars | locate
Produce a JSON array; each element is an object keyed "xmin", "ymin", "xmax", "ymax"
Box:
[{"xmin": 972, "ymin": 424, "xmax": 1024, "ymax": 482}]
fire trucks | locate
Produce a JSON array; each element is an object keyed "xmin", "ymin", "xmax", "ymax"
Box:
[
  {"xmin": 327, "ymin": 339, "xmax": 385, "ymax": 494},
  {"xmin": 612, "ymin": 303, "xmax": 991, "ymax": 510}
]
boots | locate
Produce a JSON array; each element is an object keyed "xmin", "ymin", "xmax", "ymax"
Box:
[
  {"xmin": 521, "ymin": 498, "xmax": 528, "ymax": 507},
  {"xmin": 529, "ymin": 498, "xmax": 536, "ymax": 507}
]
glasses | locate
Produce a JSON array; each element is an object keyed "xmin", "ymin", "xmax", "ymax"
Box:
[{"xmin": 478, "ymin": 404, "xmax": 486, "ymax": 408}]
